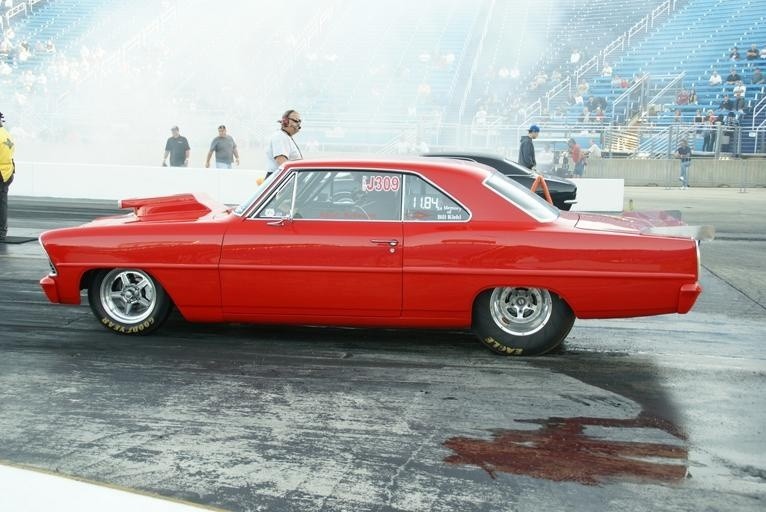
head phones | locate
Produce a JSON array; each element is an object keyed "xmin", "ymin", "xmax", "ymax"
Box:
[{"xmin": 283, "ymin": 109, "xmax": 294, "ymax": 128}]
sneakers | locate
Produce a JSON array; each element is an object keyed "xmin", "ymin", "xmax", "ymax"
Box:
[
  {"xmin": 680, "ymin": 186, "xmax": 687, "ymax": 190},
  {"xmin": 679, "ymin": 176, "xmax": 684, "ymax": 181}
]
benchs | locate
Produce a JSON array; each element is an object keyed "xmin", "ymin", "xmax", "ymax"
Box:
[{"xmin": 0, "ymin": 0, "xmax": 126, "ymax": 90}]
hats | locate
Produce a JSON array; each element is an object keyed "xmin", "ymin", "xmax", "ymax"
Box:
[{"xmin": 527, "ymin": 125, "xmax": 539, "ymax": 132}]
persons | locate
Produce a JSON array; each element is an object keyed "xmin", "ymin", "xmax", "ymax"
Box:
[
  {"xmin": 204, "ymin": 124, "xmax": 240, "ymax": 170},
  {"xmin": 263, "ymin": 108, "xmax": 304, "ymax": 185},
  {"xmin": 0, "ymin": 112, "xmax": 18, "ymax": 241},
  {"xmin": 478, "ymin": 38, "xmax": 766, "ymax": 188},
  {"xmin": 160, "ymin": 125, "xmax": 191, "ymax": 168}
]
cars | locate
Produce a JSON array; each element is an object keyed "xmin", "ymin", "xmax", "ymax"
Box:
[
  {"xmin": 414, "ymin": 148, "xmax": 580, "ymax": 213},
  {"xmin": 33, "ymin": 150, "xmax": 722, "ymax": 360}
]
